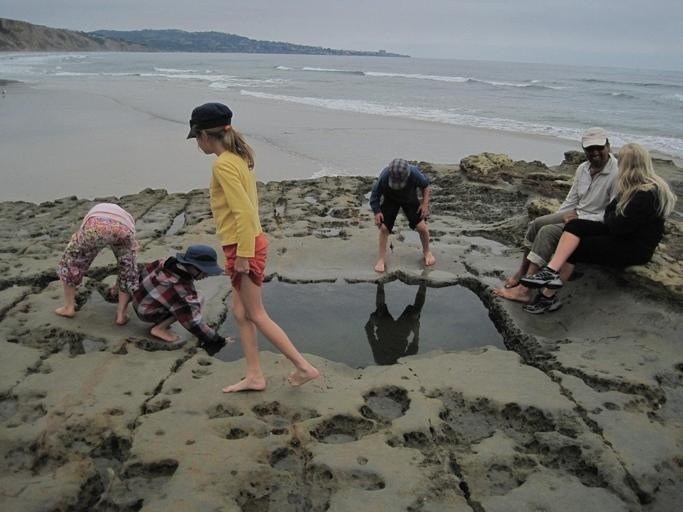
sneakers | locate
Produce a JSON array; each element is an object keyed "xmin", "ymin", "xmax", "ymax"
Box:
[
  {"xmin": 523, "ymin": 296, "xmax": 561, "ymax": 314},
  {"xmin": 520, "ymin": 268, "xmax": 563, "ymax": 289}
]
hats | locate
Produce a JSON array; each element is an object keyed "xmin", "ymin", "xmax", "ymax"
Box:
[
  {"xmin": 582, "ymin": 127, "xmax": 608, "ymax": 148},
  {"xmin": 176, "ymin": 245, "xmax": 224, "ymax": 276},
  {"xmin": 388, "ymin": 159, "xmax": 411, "ymax": 190},
  {"xmin": 186, "ymin": 103, "xmax": 232, "ymax": 140}
]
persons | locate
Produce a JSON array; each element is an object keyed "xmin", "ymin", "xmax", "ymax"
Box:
[
  {"xmin": 518, "ymin": 142, "xmax": 678, "ymax": 315},
  {"xmin": 491, "ymin": 128, "xmax": 620, "ymax": 303},
  {"xmin": 185, "ymin": 102, "xmax": 318, "ymax": 393},
  {"xmin": 52, "ymin": 202, "xmax": 140, "ymax": 325},
  {"xmin": 363, "ymin": 280, "xmax": 426, "ymax": 365},
  {"xmin": 130, "ymin": 244, "xmax": 234, "ymax": 346},
  {"xmin": 369, "ymin": 158, "xmax": 436, "ymax": 273}
]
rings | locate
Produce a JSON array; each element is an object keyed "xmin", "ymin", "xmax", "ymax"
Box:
[{"xmin": 244, "ymin": 268, "xmax": 249, "ymax": 271}]
